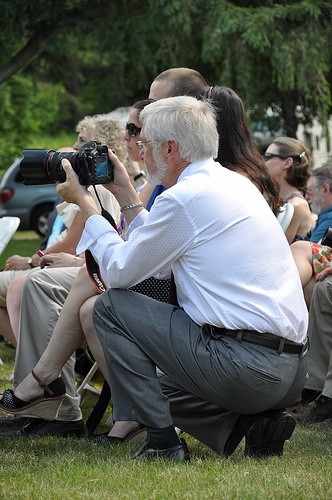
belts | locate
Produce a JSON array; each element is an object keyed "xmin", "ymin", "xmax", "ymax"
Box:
[{"xmin": 216, "ymin": 328, "xmax": 310, "ymax": 354}]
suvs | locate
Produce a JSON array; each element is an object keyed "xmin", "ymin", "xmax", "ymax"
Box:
[{"xmin": 0, "ymin": 156, "xmax": 62, "ymax": 239}]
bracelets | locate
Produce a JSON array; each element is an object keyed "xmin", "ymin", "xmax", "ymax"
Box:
[{"xmin": 118, "ymin": 202, "xmax": 143, "ymax": 212}]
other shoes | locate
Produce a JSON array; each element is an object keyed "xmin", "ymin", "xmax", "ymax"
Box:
[{"xmin": 289, "ymin": 394, "xmax": 332, "ymax": 423}]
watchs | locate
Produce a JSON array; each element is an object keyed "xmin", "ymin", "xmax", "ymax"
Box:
[
  {"xmin": 27, "ymin": 256, "xmax": 34, "ymax": 267},
  {"xmin": 133, "ymin": 170, "xmax": 146, "ymax": 181}
]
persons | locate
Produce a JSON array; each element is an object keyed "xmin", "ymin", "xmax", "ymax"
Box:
[
  {"xmin": 255, "ymin": 136, "xmax": 332, "ymax": 425},
  {"xmin": 3, "ymin": 86, "xmax": 287, "ymax": 450},
  {"xmin": 56, "ymin": 96, "xmax": 309, "ymax": 463},
  {"xmin": 0, "ymin": 67, "xmax": 209, "ymax": 437}
]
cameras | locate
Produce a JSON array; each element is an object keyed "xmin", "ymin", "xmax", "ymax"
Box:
[
  {"xmin": 19, "ymin": 140, "xmax": 115, "ymax": 186},
  {"xmin": 321, "ymin": 227, "xmax": 332, "ymax": 248}
]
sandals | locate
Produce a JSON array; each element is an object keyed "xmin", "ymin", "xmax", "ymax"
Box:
[
  {"xmin": 87, "ymin": 421, "xmax": 147, "ymax": 447},
  {"xmin": 0, "ymin": 370, "xmax": 67, "ymax": 411}
]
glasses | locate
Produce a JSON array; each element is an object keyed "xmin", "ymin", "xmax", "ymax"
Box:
[
  {"xmin": 263, "ymin": 152, "xmax": 287, "ymax": 161},
  {"xmin": 126, "ymin": 123, "xmax": 142, "ymax": 136},
  {"xmin": 136, "ymin": 140, "xmax": 159, "ymax": 150}
]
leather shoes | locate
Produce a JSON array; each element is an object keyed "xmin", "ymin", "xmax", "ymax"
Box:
[
  {"xmin": 0, "ymin": 417, "xmax": 88, "ymax": 439},
  {"xmin": 129, "ymin": 438, "xmax": 190, "ymax": 464},
  {"xmin": 243, "ymin": 410, "xmax": 297, "ymax": 460}
]
